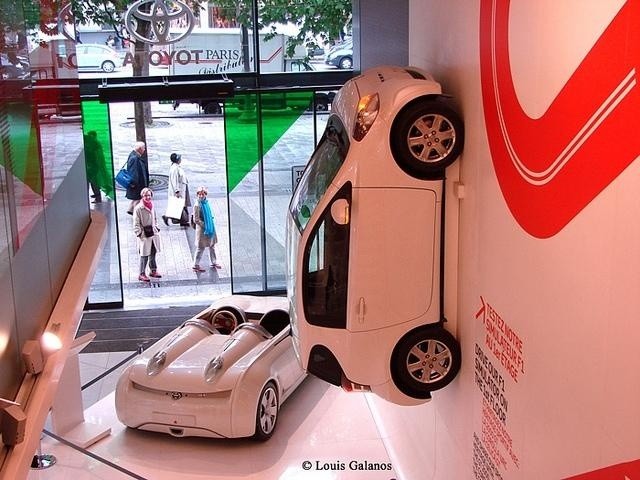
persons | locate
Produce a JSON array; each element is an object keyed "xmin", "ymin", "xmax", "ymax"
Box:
[
  {"xmin": 213, "ymin": 10, "xmax": 236, "ymax": 28},
  {"xmin": 124, "ymin": 140, "xmax": 150, "ymax": 216},
  {"xmin": 161, "ymin": 152, "xmax": 193, "ymax": 228},
  {"xmin": 191, "ymin": 185, "xmax": 223, "ymax": 273},
  {"xmin": 132, "ymin": 187, "xmax": 164, "ymax": 283},
  {"xmin": 87, "ymin": 129, "xmax": 109, "ymax": 190},
  {"xmin": 77, "ymin": 28, "xmax": 83, "ymax": 44},
  {"xmin": 84, "ymin": 134, "xmax": 108, "ymax": 205},
  {"xmin": 105, "ymin": 32, "xmax": 131, "ymax": 50}
]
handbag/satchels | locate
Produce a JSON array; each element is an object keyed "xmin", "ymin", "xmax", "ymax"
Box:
[
  {"xmin": 143, "ymin": 225, "xmax": 154, "ymax": 238},
  {"xmin": 115, "ymin": 155, "xmax": 139, "ymax": 190},
  {"xmin": 190, "ymin": 214, "xmax": 196, "ymax": 230}
]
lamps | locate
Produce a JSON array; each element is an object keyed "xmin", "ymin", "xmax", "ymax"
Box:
[{"xmin": 21, "ymin": 329, "xmax": 64, "ymax": 375}]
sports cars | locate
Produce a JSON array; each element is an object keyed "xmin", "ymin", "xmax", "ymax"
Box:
[{"xmin": 115, "ymin": 294, "xmax": 309, "ymax": 442}]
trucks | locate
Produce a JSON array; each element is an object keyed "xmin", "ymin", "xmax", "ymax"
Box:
[{"xmin": 169, "ymin": 28, "xmax": 330, "ymax": 114}]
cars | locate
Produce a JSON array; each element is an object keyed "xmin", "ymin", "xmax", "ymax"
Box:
[
  {"xmin": 284, "ymin": 65, "xmax": 463, "ymax": 406},
  {"xmin": 0, "ymin": 51, "xmax": 29, "ymax": 80},
  {"xmin": 307, "ymin": 38, "xmax": 354, "ymax": 68},
  {"xmin": 76, "ymin": 44, "xmax": 124, "ymax": 73}
]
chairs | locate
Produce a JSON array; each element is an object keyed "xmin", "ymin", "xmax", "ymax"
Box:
[
  {"xmin": 188, "ymin": 315, "xmax": 218, "ymax": 334},
  {"xmin": 237, "ymin": 321, "xmax": 271, "ymax": 339}
]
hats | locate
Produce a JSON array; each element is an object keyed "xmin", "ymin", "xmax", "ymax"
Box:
[
  {"xmin": 87, "ymin": 131, "xmax": 97, "ymax": 136},
  {"xmin": 175, "ymin": 154, "xmax": 182, "ymax": 160}
]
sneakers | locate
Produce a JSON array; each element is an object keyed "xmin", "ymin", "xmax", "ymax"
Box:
[
  {"xmin": 179, "ymin": 222, "xmax": 190, "ymax": 226},
  {"xmin": 90, "ymin": 193, "xmax": 101, "ymax": 203},
  {"xmin": 161, "ymin": 214, "xmax": 169, "ymax": 226},
  {"xmin": 210, "ymin": 263, "xmax": 222, "ymax": 269},
  {"xmin": 192, "ymin": 266, "xmax": 206, "ymax": 273},
  {"xmin": 138, "ymin": 272, "xmax": 150, "ymax": 282},
  {"xmin": 148, "ymin": 270, "xmax": 162, "ymax": 278},
  {"xmin": 127, "ymin": 211, "xmax": 133, "ymax": 215}
]
